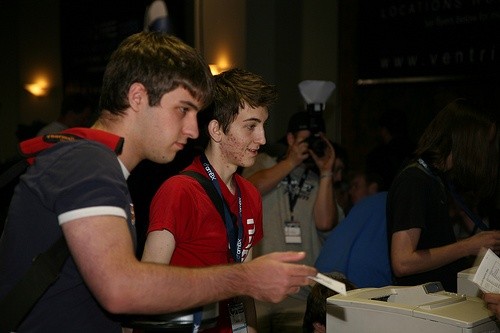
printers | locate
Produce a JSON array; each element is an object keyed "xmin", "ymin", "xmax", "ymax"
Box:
[{"xmin": 325, "ymin": 281, "xmax": 500, "ymax": 333}]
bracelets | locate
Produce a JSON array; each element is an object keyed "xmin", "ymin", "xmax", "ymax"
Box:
[{"xmin": 319, "ymin": 174, "xmax": 334, "ymax": 180}]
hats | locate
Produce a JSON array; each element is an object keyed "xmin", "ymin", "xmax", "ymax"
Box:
[{"xmin": 276, "ymin": 111, "xmax": 327, "ymax": 147}]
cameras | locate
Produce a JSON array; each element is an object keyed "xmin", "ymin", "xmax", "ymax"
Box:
[{"xmin": 303, "ymin": 128, "xmax": 327, "ymax": 156}]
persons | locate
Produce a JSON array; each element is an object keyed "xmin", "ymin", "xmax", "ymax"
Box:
[
  {"xmin": 141, "ymin": 69, "xmax": 275, "ymax": 333},
  {"xmin": 241, "ymin": 110, "xmax": 344, "ymax": 333},
  {"xmin": 302, "ymin": 120, "xmax": 395, "ymax": 333},
  {"xmin": 0, "ymin": 31, "xmax": 317, "ymax": 333},
  {"xmin": 386, "ymin": 94, "xmax": 500, "ymax": 293}
]
